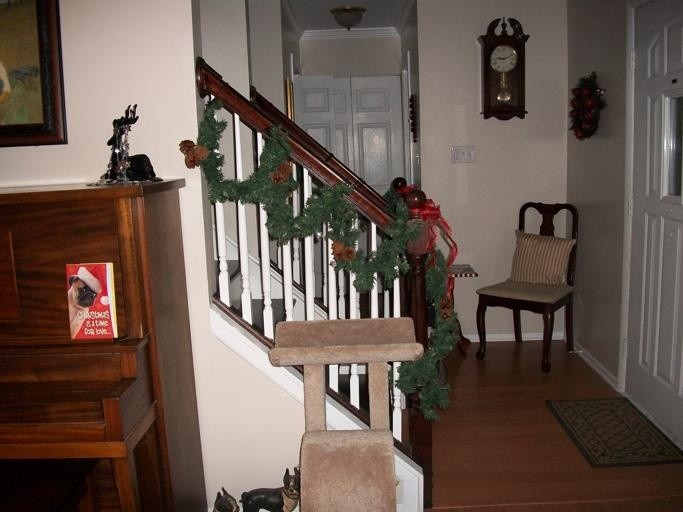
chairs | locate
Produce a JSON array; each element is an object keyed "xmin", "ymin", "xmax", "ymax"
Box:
[{"xmin": 476, "ymin": 203, "xmax": 579, "ymax": 372}]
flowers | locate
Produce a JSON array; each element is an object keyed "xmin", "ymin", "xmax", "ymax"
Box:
[{"xmin": 569, "ymin": 71, "xmax": 607, "ymax": 141}]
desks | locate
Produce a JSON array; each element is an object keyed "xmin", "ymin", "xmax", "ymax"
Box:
[{"xmin": 0, "ymin": 179, "xmax": 208, "ymax": 512}]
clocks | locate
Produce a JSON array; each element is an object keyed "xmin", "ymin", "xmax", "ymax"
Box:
[{"xmin": 480, "ymin": 17, "xmax": 530, "ymax": 121}]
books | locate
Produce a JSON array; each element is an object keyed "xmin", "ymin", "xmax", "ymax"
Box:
[{"xmin": 66, "ymin": 262, "xmax": 118, "ymax": 339}]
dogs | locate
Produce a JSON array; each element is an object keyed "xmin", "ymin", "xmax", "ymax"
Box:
[
  {"xmin": 67, "ymin": 272, "xmax": 98, "ymax": 340},
  {"xmin": 239, "ymin": 467, "xmax": 301, "ymax": 512},
  {"xmin": 211, "ymin": 486, "xmax": 239, "ymax": 512}
]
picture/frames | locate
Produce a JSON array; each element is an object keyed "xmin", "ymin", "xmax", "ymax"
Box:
[{"xmin": 0, "ymin": 0, "xmax": 67, "ymax": 147}]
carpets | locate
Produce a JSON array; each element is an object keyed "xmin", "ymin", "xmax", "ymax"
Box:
[{"xmin": 545, "ymin": 395, "xmax": 683, "ymax": 467}]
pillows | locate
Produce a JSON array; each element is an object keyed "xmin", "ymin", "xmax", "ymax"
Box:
[{"xmin": 510, "ymin": 229, "xmax": 576, "ymax": 287}]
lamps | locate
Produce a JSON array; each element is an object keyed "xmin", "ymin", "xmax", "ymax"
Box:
[{"xmin": 331, "ymin": 7, "xmax": 367, "ymax": 32}]
